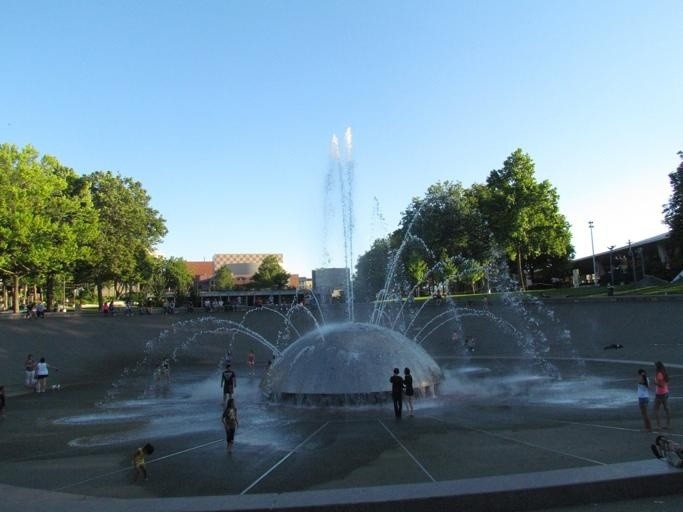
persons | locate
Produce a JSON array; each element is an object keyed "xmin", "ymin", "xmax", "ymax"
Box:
[
  {"xmin": 247, "ymin": 348, "xmax": 256, "ymax": 376},
  {"xmin": 452, "ymin": 330, "xmax": 479, "ymax": 352},
  {"xmin": 102, "ymin": 296, "xmax": 175, "ymax": 318},
  {"xmin": 403, "ymin": 367, "xmax": 415, "ymax": 418},
  {"xmin": 389, "ymin": 367, "xmax": 403, "ymax": 418},
  {"xmin": 205, "ymin": 297, "xmax": 241, "ymax": 312},
  {"xmin": 256, "ymin": 295, "xmax": 274, "ymax": 311},
  {"xmin": 221, "ymin": 398, "xmax": 239, "ymax": 454},
  {"xmin": 26, "ymin": 354, "xmax": 58, "ymax": 393},
  {"xmin": 0, "ymin": 384, "xmax": 7, "ymax": 421},
  {"xmin": 153, "ymin": 357, "xmax": 171, "ymax": 385},
  {"xmin": 221, "ymin": 364, "xmax": 236, "ymax": 402},
  {"xmin": 653, "ymin": 360, "xmax": 673, "ymax": 431},
  {"xmin": 130, "ymin": 442, "xmax": 156, "ymax": 482},
  {"xmin": 24, "ymin": 299, "xmax": 58, "ymax": 320},
  {"xmin": 469, "ymin": 295, "xmax": 487, "ymax": 312},
  {"xmin": 437, "ymin": 291, "xmax": 448, "ymax": 304},
  {"xmin": 636, "ymin": 367, "xmax": 653, "ymax": 434}
]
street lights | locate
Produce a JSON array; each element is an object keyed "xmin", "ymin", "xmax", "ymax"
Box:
[{"xmin": 587, "ymin": 221, "xmax": 597, "ymax": 286}]
